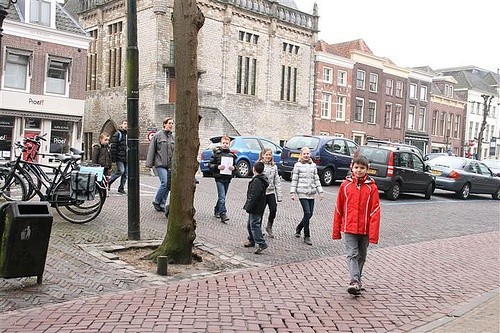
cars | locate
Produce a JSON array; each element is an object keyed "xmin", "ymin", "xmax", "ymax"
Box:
[
  {"xmin": 428, "ymin": 153, "xmax": 449, "ymax": 162},
  {"xmin": 429, "ymin": 157, "xmax": 500, "ymax": 201},
  {"xmin": 481, "ymin": 159, "xmax": 500, "ymax": 176}
]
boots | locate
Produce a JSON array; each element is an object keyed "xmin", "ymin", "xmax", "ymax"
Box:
[
  {"xmin": 262, "ymin": 233, "xmax": 266, "ymax": 236},
  {"xmin": 266, "ymin": 222, "xmax": 274, "ymax": 238}
]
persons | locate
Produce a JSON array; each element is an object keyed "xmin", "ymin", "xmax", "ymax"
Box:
[
  {"xmin": 332, "ymin": 156, "xmax": 380, "ymax": 294},
  {"xmin": 290, "ymin": 147, "xmax": 324, "ymax": 244},
  {"xmin": 256, "ymin": 148, "xmax": 282, "ymax": 238},
  {"xmin": 92, "ymin": 131, "xmax": 111, "ymax": 185},
  {"xmin": 108, "ymin": 120, "xmax": 129, "ymax": 194},
  {"xmin": 208, "ymin": 136, "xmax": 237, "ymax": 222},
  {"xmin": 243, "ymin": 162, "xmax": 269, "ymax": 254},
  {"xmin": 146, "ymin": 118, "xmax": 174, "ymax": 212},
  {"xmin": 165, "ymin": 115, "xmax": 203, "ymax": 218}
]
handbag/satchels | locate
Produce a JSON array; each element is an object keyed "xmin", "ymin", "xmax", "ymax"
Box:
[{"xmin": 69, "ymin": 167, "xmax": 108, "ymax": 201}]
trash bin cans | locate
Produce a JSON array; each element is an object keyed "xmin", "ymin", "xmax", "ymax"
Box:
[{"xmin": 0, "ymin": 201, "xmax": 54, "ymax": 278}]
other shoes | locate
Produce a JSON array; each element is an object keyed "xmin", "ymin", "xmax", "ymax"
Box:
[
  {"xmin": 304, "ymin": 237, "xmax": 312, "ymax": 245},
  {"xmin": 108, "ymin": 182, "xmax": 110, "ymax": 191},
  {"xmin": 153, "ymin": 202, "xmax": 161, "ymax": 211},
  {"xmin": 347, "ymin": 282, "xmax": 359, "ymax": 297},
  {"xmin": 255, "ymin": 244, "xmax": 267, "ymax": 254},
  {"xmin": 118, "ymin": 187, "xmax": 126, "ymax": 194},
  {"xmin": 160, "ymin": 207, "xmax": 166, "ymax": 212},
  {"xmin": 214, "ymin": 207, "xmax": 220, "ymax": 218},
  {"xmin": 220, "ymin": 212, "xmax": 230, "ymax": 222},
  {"xmin": 244, "ymin": 241, "xmax": 255, "ymax": 247},
  {"xmin": 295, "ymin": 226, "xmax": 301, "ymax": 238}
]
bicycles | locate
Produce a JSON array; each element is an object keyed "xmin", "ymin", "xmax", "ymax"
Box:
[{"xmin": 0, "ymin": 132, "xmax": 110, "ymax": 224}]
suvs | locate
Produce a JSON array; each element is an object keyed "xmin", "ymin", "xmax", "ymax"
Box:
[
  {"xmin": 346, "ymin": 139, "xmax": 437, "ymax": 201},
  {"xmin": 199, "ymin": 136, "xmax": 284, "ymax": 178},
  {"xmin": 279, "ymin": 134, "xmax": 361, "ymax": 187}
]
